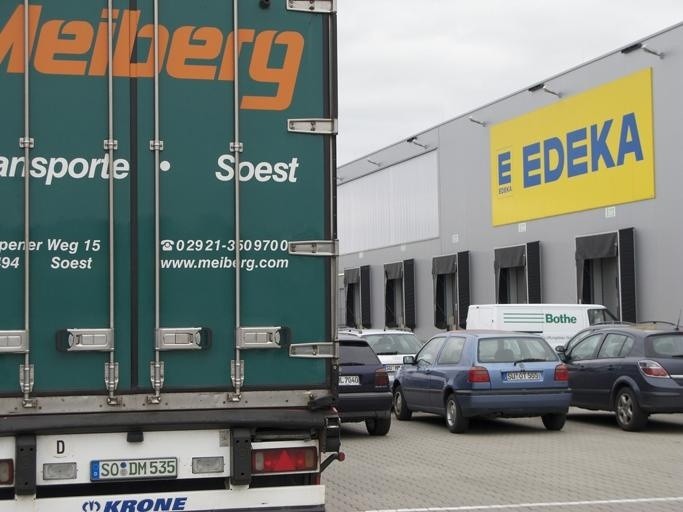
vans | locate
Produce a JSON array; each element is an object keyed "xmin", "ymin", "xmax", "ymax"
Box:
[{"xmin": 464, "ymin": 302, "xmax": 621, "ymax": 354}]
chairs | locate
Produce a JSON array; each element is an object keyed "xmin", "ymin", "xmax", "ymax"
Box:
[
  {"xmin": 658, "ymin": 343, "xmax": 674, "ymax": 354},
  {"xmin": 496, "ymin": 349, "xmax": 513, "ymax": 362}
]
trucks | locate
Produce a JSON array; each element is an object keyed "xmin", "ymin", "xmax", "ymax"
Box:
[{"xmin": 0, "ymin": 0, "xmax": 346, "ymax": 507}]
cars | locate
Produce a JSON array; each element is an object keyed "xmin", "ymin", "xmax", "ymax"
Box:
[
  {"xmin": 332, "ymin": 331, "xmax": 395, "ymax": 436},
  {"xmin": 360, "ymin": 329, "xmax": 572, "ymax": 434},
  {"xmin": 558, "ymin": 320, "xmax": 683, "ymax": 430}
]
